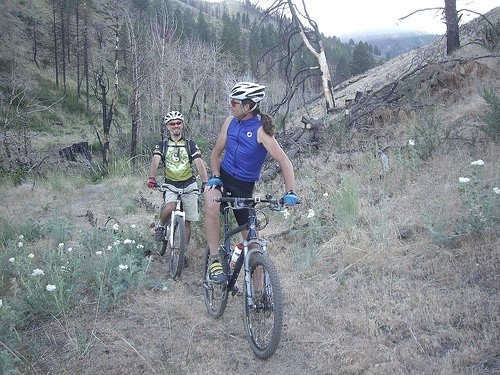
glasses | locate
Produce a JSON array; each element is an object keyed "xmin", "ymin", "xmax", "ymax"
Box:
[
  {"xmin": 166, "ymin": 122, "xmax": 182, "ymax": 126},
  {"xmin": 231, "ymin": 101, "xmax": 240, "ymax": 107}
]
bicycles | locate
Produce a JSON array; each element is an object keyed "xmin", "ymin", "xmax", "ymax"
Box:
[
  {"xmin": 148, "ymin": 181, "xmax": 204, "ymax": 282},
  {"xmin": 202, "ymin": 192, "xmax": 301, "ymax": 360}
]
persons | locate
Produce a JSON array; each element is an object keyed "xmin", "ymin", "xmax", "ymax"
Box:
[
  {"xmin": 202, "ymin": 81, "xmax": 304, "ymax": 313},
  {"xmin": 145, "ymin": 110, "xmax": 209, "ymax": 268}
]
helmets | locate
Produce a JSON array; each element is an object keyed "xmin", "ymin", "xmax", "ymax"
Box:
[
  {"xmin": 229, "ymin": 82, "xmax": 267, "ymax": 103},
  {"xmin": 164, "ymin": 111, "xmax": 184, "ymax": 124}
]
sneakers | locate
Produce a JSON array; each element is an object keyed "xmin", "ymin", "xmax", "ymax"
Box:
[
  {"xmin": 208, "ymin": 256, "xmax": 227, "ymax": 283},
  {"xmin": 184, "ymin": 256, "xmax": 188, "ymax": 267},
  {"xmin": 155, "ymin": 226, "xmax": 164, "ymax": 242},
  {"xmin": 254, "ymin": 291, "xmax": 273, "ymax": 309}
]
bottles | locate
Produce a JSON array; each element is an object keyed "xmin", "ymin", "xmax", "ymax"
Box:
[{"xmin": 229, "ymin": 243, "xmax": 244, "ymax": 262}]
